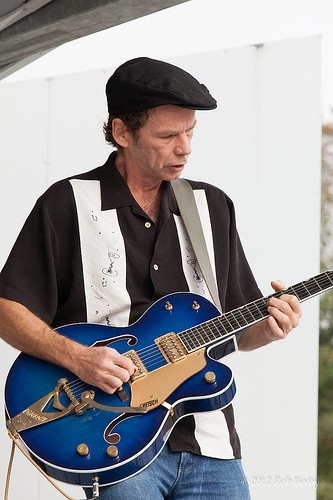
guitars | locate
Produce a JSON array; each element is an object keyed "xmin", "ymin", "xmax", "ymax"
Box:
[{"xmin": 4, "ymin": 269, "xmax": 333, "ymax": 487}]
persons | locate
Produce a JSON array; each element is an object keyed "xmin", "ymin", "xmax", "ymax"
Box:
[{"xmin": 0, "ymin": 57, "xmax": 304, "ymax": 500}]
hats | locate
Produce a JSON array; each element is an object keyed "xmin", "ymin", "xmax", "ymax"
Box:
[{"xmin": 106, "ymin": 57, "xmax": 217, "ymax": 114}]
